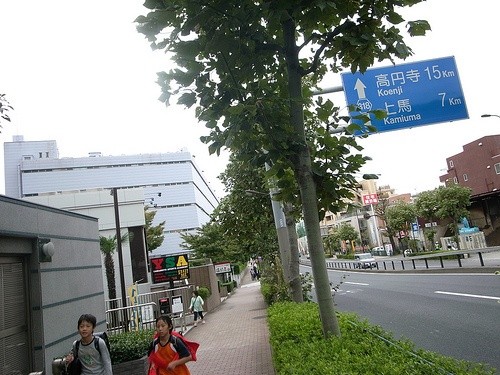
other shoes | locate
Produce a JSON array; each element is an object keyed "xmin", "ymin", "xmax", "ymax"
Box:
[
  {"xmin": 192, "ymin": 321, "xmax": 197, "ymax": 327},
  {"xmin": 201, "ymin": 320, "xmax": 206, "ymax": 324}
]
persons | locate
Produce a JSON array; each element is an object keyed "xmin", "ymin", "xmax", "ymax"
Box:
[
  {"xmin": 147, "ymin": 316, "xmax": 192, "ymax": 375},
  {"xmin": 65, "ymin": 314, "xmax": 113, "ymax": 375},
  {"xmin": 188, "ymin": 291, "xmax": 205, "ymax": 326},
  {"xmin": 250, "ymin": 266, "xmax": 261, "ymax": 281}
]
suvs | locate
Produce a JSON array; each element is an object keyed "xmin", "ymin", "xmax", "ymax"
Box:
[{"xmin": 353, "ymin": 253, "xmax": 377, "ymax": 269}]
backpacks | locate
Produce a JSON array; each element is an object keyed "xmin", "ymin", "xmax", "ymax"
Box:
[{"xmin": 74, "ymin": 332, "xmax": 111, "ymax": 365}]
